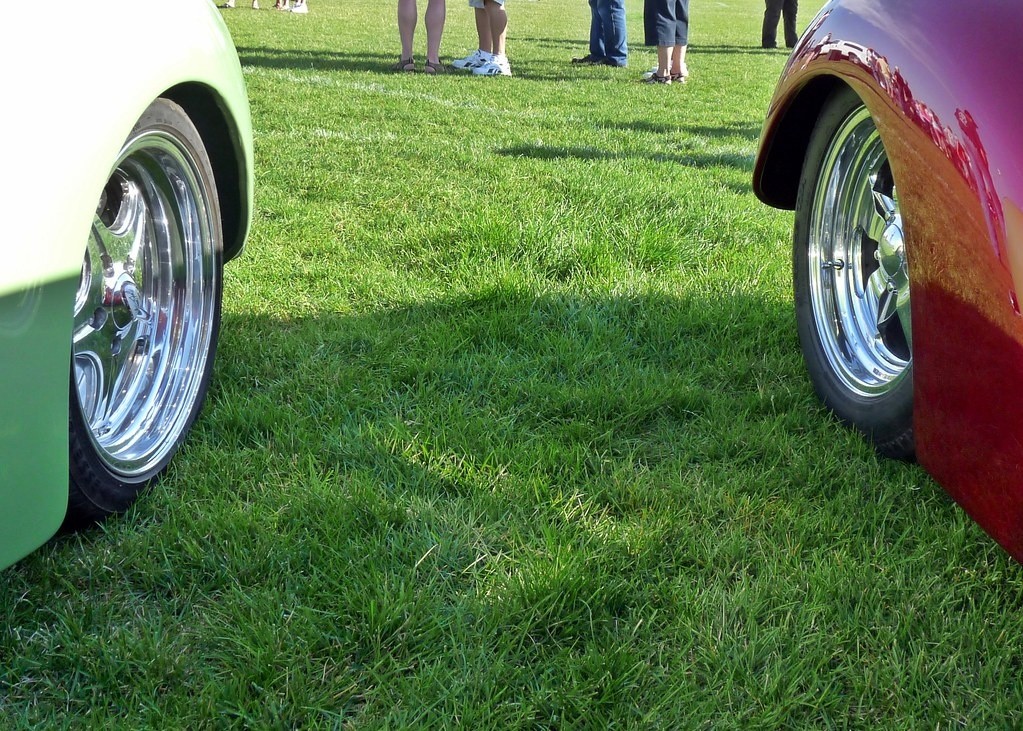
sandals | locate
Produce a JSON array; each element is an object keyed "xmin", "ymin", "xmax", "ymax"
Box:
[
  {"xmin": 425, "ymin": 59, "xmax": 455, "ymax": 74},
  {"xmin": 392, "ymin": 57, "xmax": 416, "ymax": 72}
]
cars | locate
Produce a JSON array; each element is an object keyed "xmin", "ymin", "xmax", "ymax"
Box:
[
  {"xmin": 753, "ymin": 0, "xmax": 1023, "ymax": 566},
  {"xmin": 0, "ymin": 0, "xmax": 255, "ymax": 571}
]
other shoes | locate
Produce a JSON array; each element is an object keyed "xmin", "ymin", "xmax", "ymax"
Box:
[
  {"xmin": 276, "ymin": 2, "xmax": 289, "ymax": 11},
  {"xmin": 252, "ymin": 1, "xmax": 259, "ymax": 9},
  {"xmin": 572, "ymin": 55, "xmax": 626, "ymax": 68},
  {"xmin": 224, "ymin": 2, "xmax": 234, "ymax": 7}
]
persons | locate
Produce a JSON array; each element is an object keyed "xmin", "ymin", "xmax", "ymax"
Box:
[
  {"xmin": 572, "ymin": 0, "xmax": 628, "ymax": 68},
  {"xmin": 766, "ymin": 9, "xmax": 832, "ymax": 118},
  {"xmin": 762, "ymin": 0, "xmax": 799, "ymax": 49},
  {"xmin": 642, "ymin": 0, "xmax": 689, "ymax": 85},
  {"xmin": 225, "ymin": 0, "xmax": 308, "ymax": 13},
  {"xmin": 391, "ymin": 0, "xmax": 457, "ymax": 74},
  {"xmin": 866, "ymin": 45, "xmax": 1007, "ymax": 262},
  {"xmin": 452, "ymin": 0, "xmax": 512, "ymax": 76}
]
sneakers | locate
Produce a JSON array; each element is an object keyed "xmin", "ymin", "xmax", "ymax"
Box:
[
  {"xmin": 452, "ymin": 49, "xmax": 491, "ymax": 70},
  {"xmin": 643, "ymin": 67, "xmax": 687, "ymax": 85},
  {"xmin": 288, "ymin": 2, "xmax": 308, "ymax": 13},
  {"xmin": 473, "ymin": 55, "xmax": 512, "ymax": 76}
]
